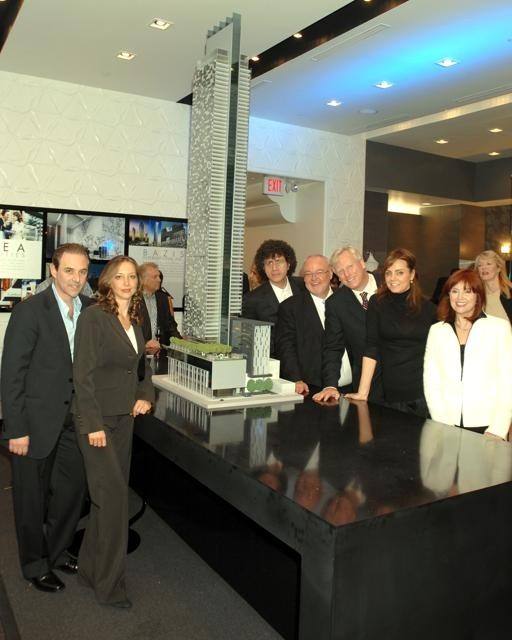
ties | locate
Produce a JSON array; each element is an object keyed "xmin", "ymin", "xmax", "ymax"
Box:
[{"xmin": 361, "ymin": 292, "xmax": 369, "ymax": 311}]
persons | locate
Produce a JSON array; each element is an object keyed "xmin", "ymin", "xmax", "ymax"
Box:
[
  {"xmin": 247, "ymin": 397, "xmax": 511, "ymax": 527},
  {"xmin": 1, "ymin": 209, "xmax": 13, "ymax": 240},
  {"xmin": 1, "ymin": 241, "xmax": 91, "ymax": 595},
  {"xmin": 241, "ymin": 239, "xmax": 512, "ymax": 444},
  {"xmin": 4, "ymin": 211, "xmax": 26, "ymax": 241},
  {"xmin": 133, "ymin": 262, "xmax": 181, "ymax": 384},
  {"xmin": 68, "ymin": 253, "xmax": 156, "ymax": 610}
]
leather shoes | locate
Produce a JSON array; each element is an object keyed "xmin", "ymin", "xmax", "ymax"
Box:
[
  {"xmin": 112, "ymin": 599, "xmax": 131, "ymax": 608},
  {"xmin": 56, "ymin": 559, "xmax": 78, "ymax": 574},
  {"xmin": 27, "ymin": 571, "xmax": 64, "ymax": 592}
]
